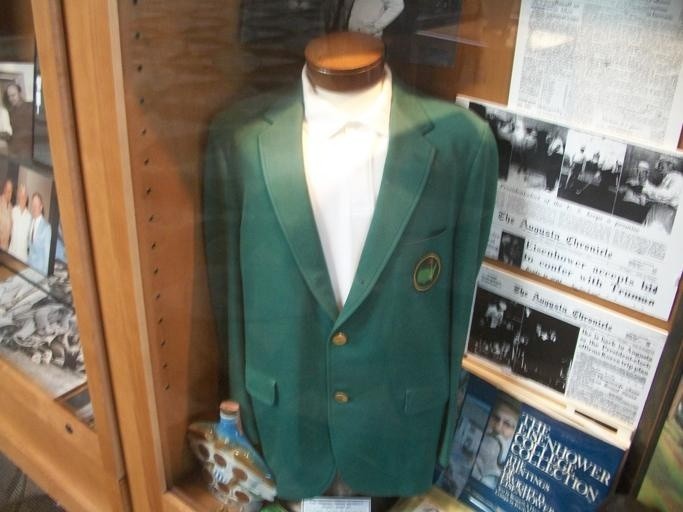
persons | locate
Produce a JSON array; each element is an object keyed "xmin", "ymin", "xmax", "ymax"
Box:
[
  {"xmin": 204, "ymin": 29, "xmax": 500, "ymax": 507},
  {"xmin": 487, "ymin": 402, "xmax": 518, "ymax": 438},
  {"xmin": 516, "ymin": 129, "xmax": 540, "ymax": 176},
  {"xmin": 563, "ymin": 146, "xmax": 587, "ymax": 193},
  {"xmin": 0, "ymin": 179, "xmax": 13, "ymax": 251},
  {"xmin": 629, "ymin": 154, "xmax": 683, "ymax": 233},
  {"xmin": 469, "ymin": 299, "xmax": 576, "ymax": 392},
  {"xmin": 543, "ymin": 131, "xmax": 563, "ymax": 194},
  {"xmin": 25, "ymin": 192, "xmax": 52, "ymax": 272},
  {"xmin": 585, "ymin": 150, "xmax": 601, "ymax": 172},
  {"xmin": 5, "ymin": 82, "xmax": 36, "ymax": 155},
  {"xmin": 0, "ymin": 94, "xmax": 13, "ymax": 156},
  {"xmin": 616, "ymin": 159, "xmax": 654, "ymax": 205},
  {"xmin": 9, "ymin": 183, "xmax": 32, "ymax": 261}
]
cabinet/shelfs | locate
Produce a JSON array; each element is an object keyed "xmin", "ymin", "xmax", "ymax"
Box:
[{"xmin": 0, "ymin": 0, "xmax": 167, "ymax": 510}]
